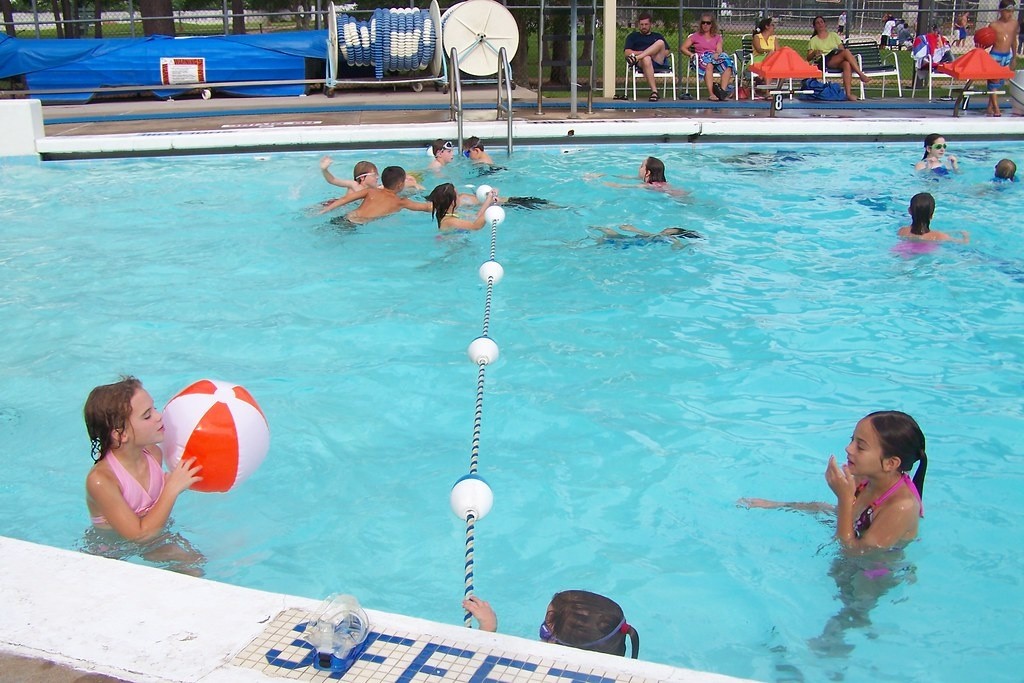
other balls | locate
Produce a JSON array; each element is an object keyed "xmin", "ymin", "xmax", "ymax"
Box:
[
  {"xmin": 159, "ymin": 377, "xmax": 273, "ymax": 493},
  {"xmin": 975, "ymin": 27, "xmax": 995, "ymax": 47}
]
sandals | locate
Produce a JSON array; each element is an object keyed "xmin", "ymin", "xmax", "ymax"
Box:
[
  {"xmin": 626, "ymin": 56, "xmax": 639, "ymax": 67},
  {"xmin": 648, "ymin": 91, "xmax": 659, "ymax": 102}
]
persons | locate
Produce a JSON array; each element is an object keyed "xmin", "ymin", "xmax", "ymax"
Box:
[
  {"xmin": 880, "ymin": 11, "xmax": 969, "ymax": 50},
  {"xmin": 1016, "ymin": 4, "xmax": 1024, "ymax": 55},
  {"xmin": 681, "ymin": 12, "xmax": 733, "ymax": 102},
  {"xmin": 753, "ymin": 18, "xmax": 780, "ymax": 100},
  {"xmin": 326, "ymin": 167, "xmax": 432, "ymax": 218},
  {"xmin": 757, "ymin": 10, "xmax": 781, "ymax": 24},
  {"xmin": 423, "ymin": 183, "xmax": 498, "ymax": 231},
  {"xmin": 915, "ymin": 133, "xmax": 957, "ymax": 171},
  {"xmin": 898, "ymin": 192, "xmax": 948, "ymax": 240},
  {"xmin": 990, "ymin": 159, "xmax": 1017, "ymax": 182},
  {"xmin": 837, "ymin": 11, "xmax": 847, "ymax": 37},
  {"xmin": 973, "ymin": 0, "xmax": 1019, "ymax": 118},
  {"xmin": 807, "ymin": 16, "xmax": 872, "ymax": 102},
  {"xmin": 320, "ymin": 155, "xmax": 418, "ymax": 194},
  {"xmin": 624, "ymin": 12, "xmax": 671, "ymax": 102},
  {"xmin": 639, "ymin": 156, "xmax": 667, "ymax": 187},
  {"xmin": 463, "ymin": 136, "xmax": 492, "ymax": 163},
  {"xmin": 84, "ymin": 377, "xmax": 204, "ymax": 545},
  {"xmin": 461, "ymin": 589, "xmax": 640, "ymax": 659},
  {"xmin": 431, "ymin": 139, "xmax": 455, "ymax": 166},
  {"xmin": 826, "ymin": 411, "xmax": 927, "ymax": 548}
]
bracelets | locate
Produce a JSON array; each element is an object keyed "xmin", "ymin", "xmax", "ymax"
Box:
[{"xmin": 715, "ymin": 52, "xmax": 720, "ymax": 55}]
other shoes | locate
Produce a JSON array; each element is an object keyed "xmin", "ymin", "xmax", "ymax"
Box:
[
  {"xmin": 753, "ymin": 94, "xmax": 773, "ymax": 101},
  {"xmin": 993, "ymin": 113, "xmax": 1002, "ymax": 117},
  {"xmin": 614, "ymin": 95, "xmax": 628, "ymax": 100},
  {"xmin": 678, "ymin": 92, "xmax": 695, "ymax": 100}
]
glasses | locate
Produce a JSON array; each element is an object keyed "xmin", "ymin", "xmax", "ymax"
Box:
[
  {"xmin": 928, "ymin": 143, "xmax": 947, "ymax": 150},
  {"xmin": 701, "ymin": 20, "xmax": 712, "ymax": 26},
  {"xmin": 537, "ymin": 620, "xmax": 558, "ymax": 644},
  {"xmin": 355, "ymin": 171, "xmax": 380, "ymax": 180},
  {"xmin": 434, "ymin": 140, "xmax": 454, "ymax": 158},
  {"xmin": 313, "ymin": 593, "xmax": 370, "ymax": 671},
  {"xmin": 463, "ymin": 142, "xmax": 479, "ymax": 159},
  {"xmin": 999, "ymin": 3, "xmax": 1021, "ymax": 11}
]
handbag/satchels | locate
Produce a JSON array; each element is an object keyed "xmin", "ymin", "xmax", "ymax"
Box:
[
  {"xmin": 738, "ymin": 84, "xmax": 751, "ymax": 100},
  {"xmin": 712, "ymin": 82, "xmax": 728, "ymax": 101},
  {"xmin": 798, "ymin": 76, "xmax": 847, "ymax": 101}
]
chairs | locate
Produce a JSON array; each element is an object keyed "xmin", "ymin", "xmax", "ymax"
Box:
[
  {"xmin": 686, "ymin": 34, "xmax": 739, "ymax": 101},
  {"xmin": 623, "ymin": 52, "xmax": 676, "ymax": 101},
  {"xmin": 844, "ymin": 36, "xmax": 902, "ymax": 100},
  {"xmin": 910, "ymin": 34, "xmax": 975, "ymax": 103},
  {"xmin": 807, "ymin": 37, "xmax": 864, "ymax": 100},
  {"xmin": 740, "ymin": 34, "xmax": 790, "ymax": 101}
]
